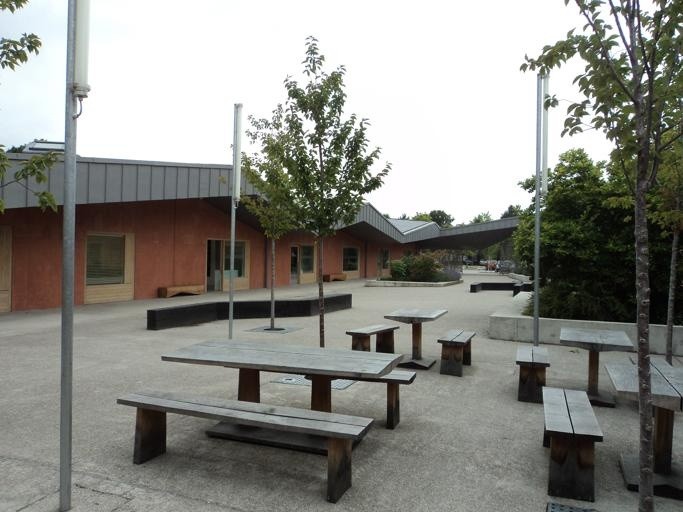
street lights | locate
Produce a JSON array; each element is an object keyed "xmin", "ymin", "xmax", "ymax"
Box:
[
  {"xmin": 230, "ymin": 104, "xmax": 244, "ymax": 338},
  {"xmin": 534, "ymin": 70, "xmax": 548, "ymax": 345},
  {"xmin": 59, "ymin": 0, "xmax": 92, "ymax": 510}
]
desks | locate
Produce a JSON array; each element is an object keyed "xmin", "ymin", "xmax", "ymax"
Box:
[
  {"xmin": 558, "ymin": 326, "xmax": 635, "ymax": 407},
  {"xmin": 601, "ymin": 355, "xmax": 683, "ymax": 500},
  {"xmin": 384, "ymin": 308, "xmax": 447, "ymax": 372},
  {"xmin": 160, "ymin": 337, "xmax": 403, "ymax": 457}
]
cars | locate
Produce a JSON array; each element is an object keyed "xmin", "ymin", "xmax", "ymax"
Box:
[{"xmin": 463, "ymin": 255, "xmax": 513, "ymax": 273}]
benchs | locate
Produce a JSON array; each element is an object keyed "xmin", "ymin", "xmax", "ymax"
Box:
[
  {"xmin": 376, "ymin": 368, "xmax": 417, "ymax": 430},
  {"xmin": 116, "ymin": 389, "xmax": 373, "ymax": 504},
  {"xmin": 345, "ymin": 323, "xmax": 400, "ymax": 354},
  {"xmin": 436, "ymin": 328, "xmax": 475, "ymax": 376},
  {"xmin": 515, "ymin": 344, "xmax": 603, "ymax": 503}
]
